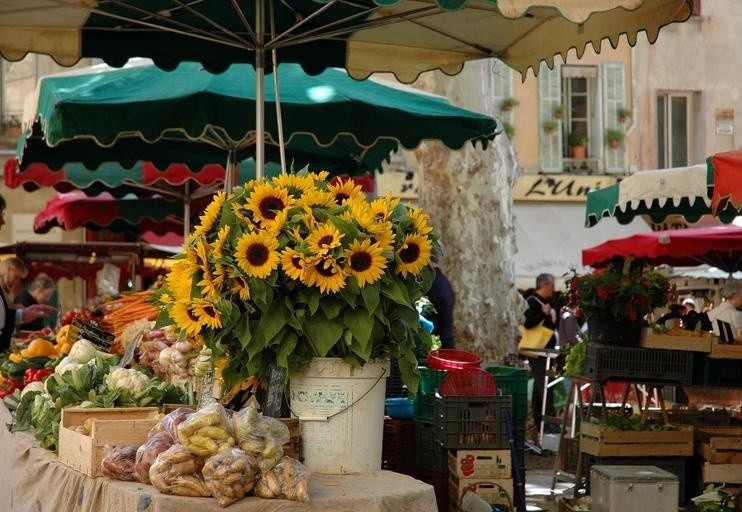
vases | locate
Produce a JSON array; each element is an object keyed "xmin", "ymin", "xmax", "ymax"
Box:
[{"xmin": 587, "ymin": 311, "xmax": 644, "ymax": 347}]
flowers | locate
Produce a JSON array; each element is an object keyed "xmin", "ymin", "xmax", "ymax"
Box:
[
  {"xmin": 561, "ymin": 261, "xmax": 678, "ymax": 320},
  {"xmin": 141, "ymin": 169, "xmax": 445, "ymax": 401}
]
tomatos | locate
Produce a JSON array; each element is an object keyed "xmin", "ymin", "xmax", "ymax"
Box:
[{"xmin": 460, "ymin": 455, "xmax": 477, "ymax": 491}]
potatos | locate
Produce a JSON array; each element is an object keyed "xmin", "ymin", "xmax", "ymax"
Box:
[{"xmin": 101, "ymin": 406, "xmax": 308, "ymax": 508}]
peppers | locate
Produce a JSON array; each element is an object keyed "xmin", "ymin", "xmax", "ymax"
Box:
[{"xmin": 0, "ymin": 306, "xmax": 112, "ymax": 399}]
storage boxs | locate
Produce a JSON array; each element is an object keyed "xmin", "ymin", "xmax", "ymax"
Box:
[{"xmin": 448, "ymin": 450, "xmax": 516, "ymax": 512}]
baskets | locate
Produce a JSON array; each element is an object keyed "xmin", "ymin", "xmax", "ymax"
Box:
[{"xmin": 581, "ymin": 339, "xmax": 694, "ymax": 384}]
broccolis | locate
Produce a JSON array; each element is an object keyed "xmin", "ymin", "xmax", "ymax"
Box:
[{"xmin": 22, "ymin": 340, "xmax": 149, "ymax": 451}]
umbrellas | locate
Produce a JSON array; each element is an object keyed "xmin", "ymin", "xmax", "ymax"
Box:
[
  {"xmin": 580, "ymin": 223, "xmax": 742, "ymax": 278},
  {"xmin": 575, "ymin": 146, "xmax": 742, "ymax": 225}
]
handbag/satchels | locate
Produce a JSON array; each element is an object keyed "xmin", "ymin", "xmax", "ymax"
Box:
[{"xmin": 516, "ymin": 325, "xmax": 554, "ymax": 359}]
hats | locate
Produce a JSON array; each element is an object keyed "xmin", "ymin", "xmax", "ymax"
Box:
[
  {"xmin": 669, "ymin": 304, "xmax": 685, "ymax": 309},
  {"xmin": 682, "ymin": 298, "xmax": 696, "ymax": 308}
]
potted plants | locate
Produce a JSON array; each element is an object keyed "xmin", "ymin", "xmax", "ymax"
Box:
[
  {"xmin": 607, "ymin": 128, "xmax": 625, "ymax": 149},
  {"xmin": 499, "ymin": 98, "xmax": 520, "ymax": 114},
  {"xmin": 543, "ymin": 121, "xmax": 558, "ymax": 134},
  {"xmin": 568, "ymin": 130, "xmax": 589, "ymax": 159},
  {"xmin": 553, "ymin": 106, "xmax": 563, "ymax": 118},
  {"xmin": 618, "ymin": 109, "xmax": 632, "ymax": 123}
]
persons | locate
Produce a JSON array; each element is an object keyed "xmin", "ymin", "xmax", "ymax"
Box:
[
  {"xmin": 0, "ymin": 195, "xmax": 59, "ymax": 353},
  {"xmin": 516, "ymin": 274, "xmax": 590, "ymax": 434},
  {"xmin": 424, "ymin": 237, "xmax": 457, "ymax": 350},
  {"xmin": 0, "ymin": 251, "xmax": 28, "ymax": 305},
  {"xmin": 12, "ymin": 273, "xmax": 57, "ymax": 331},
  {"xmin": 649, "ymin": 276, "xmax": 742, "ymax": 348}
]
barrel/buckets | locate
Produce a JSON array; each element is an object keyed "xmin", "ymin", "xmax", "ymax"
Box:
[{"xmin": 283, "ymin": 356, "xmax": 392, "ymax": 476}]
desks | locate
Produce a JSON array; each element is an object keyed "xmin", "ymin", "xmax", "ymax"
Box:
[
  {"xmin": 548, "ymin": 373, "xmax": 742, "ymax": 504},
  {"xmin": 1, "ymin": 400, "xmax": 438, "ymax": 511}
]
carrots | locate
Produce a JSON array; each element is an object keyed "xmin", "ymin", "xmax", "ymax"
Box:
[{"xmin": 104, "ymin": 289, "xmax": 161, "ymax": 335}]
